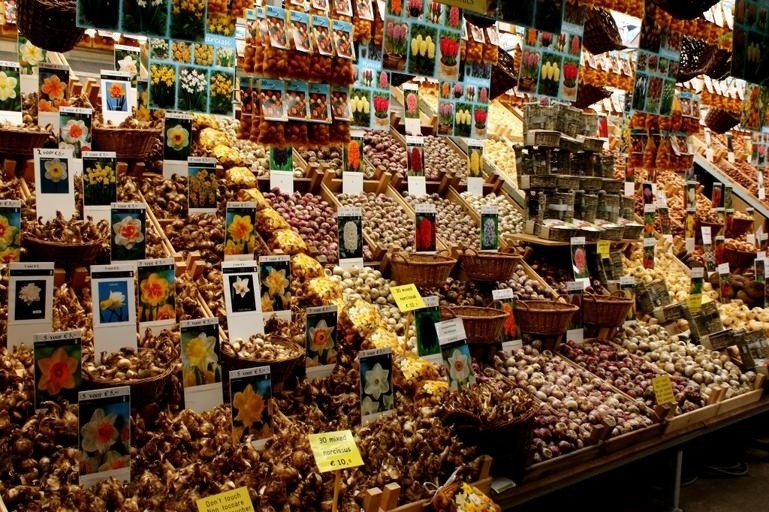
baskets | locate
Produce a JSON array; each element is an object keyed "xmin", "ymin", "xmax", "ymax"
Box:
[
  {"xmin": 695, "ymin": 221, "xmax": 724, "ymax": 245},
  {"xmin": 678, "ymin": 37, "xmax": 718, "ymax": 82},
  {"xmin": 15, "ymin": 0, "xmax": 85, "ymax": 52},
  {"xmin": 490, "ymin": 45, "xmax": 518, "ymax": 101},
  {"xmin": 725, "ymin": 219, "xmax": 753, "ymax": 238},
  {"xmin": 705, "ymin": 107, "xmax": 740, "ymax": 134},
  {"xmin": 693, "ymin": 218, "xmax": 723, "ymax": 245},
  {"xmin": 443, "ymin": 382, "xmax": 541, "ymax": 455},
  {"xmin": 82, "ymin": 347, "xmax": 181, "ymax": 403},
  {"xmin": 440, "ymin": 299, "xmax": 510, "ymax": 341},
  {"xmin": 573, "ymin": 290, "xmax": 634, "ymax": 326},
  {"xmin": 583, "ymin": 8, "xmax": 627, "ymax": 55},
  {"xmin": 386, "ymin": 248, "xmax": 457, "ymax": 287},
  {"xmin": 221, "ymin": 335, "xmax": 305, "ymax": 383},
  {"xmin": 576, "ymin": 83, "xmax": 613, "ymax": 108},
  {"xmin": 704, "ymin": 49, "xmax": 732, "ymax": 81},
  {"xmin": 451, "ymin": 245, "xmax": 524, "ymax": 284},
  {"xmin": 513, "ymin": 295, "xmax": 580, "ymax": 334},
  {"xmin": 464, "ymin": 3, "xmax": 497, "ymax": 28},
  {"xmin": 92, "ymin": 125, "xmax": 162, "ymax": 160},
  {"xmin": 23, "ymin": 231, "xmax": 108, "ymax": 265},
  {"xmin": 652, "ymin": 0, "xmax": 720, "ymax": 20},
  {"xmin": 724, "ymin": 245, "xmax": 757, "ymax": 275},
  {"xmin": 1, "ymin": 122, "xmax": 55, "ymax": 160}
]
